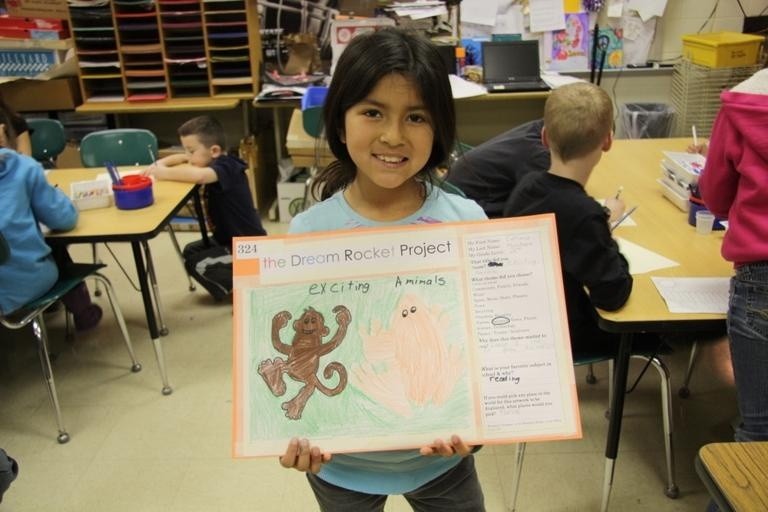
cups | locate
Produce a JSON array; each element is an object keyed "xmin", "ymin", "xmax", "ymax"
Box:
[{"xmin": 695, "ymin": 210, "xmax": 715, "ymax": 234}]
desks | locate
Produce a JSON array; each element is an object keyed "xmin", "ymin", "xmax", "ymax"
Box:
[
  {"xmin": 254, "ymin": 90, "xmax": 552, "ymax": 221},
  {"xmin": 694, "ymin": 436, "xmax": 768, "ymax": 512},
  {"xmin": 549, "ymin": 137, "xmax": 733, "ymax": 512},
  {"xmin": 8, "ymin": 163, "xmax": 200, "ymax": 394}
]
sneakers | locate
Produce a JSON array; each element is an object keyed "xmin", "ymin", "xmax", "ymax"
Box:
[{"xmin": 73, "ymin": 305, "xmax": 104, "ymax": 331}]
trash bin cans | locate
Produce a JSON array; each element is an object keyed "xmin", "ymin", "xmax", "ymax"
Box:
[{"xmin": 619, "ymin": 101, "xmax": 677, "ymax": 140}]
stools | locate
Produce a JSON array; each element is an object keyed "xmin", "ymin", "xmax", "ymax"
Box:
[{"xmin": 508, "ymin": 346, "xmax": 679, "ymax": 512}]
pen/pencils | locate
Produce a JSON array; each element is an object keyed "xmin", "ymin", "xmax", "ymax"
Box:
[
  {"xmin": 54, "ymin": 184, "xmax": 59, "ymax": 189},
  {"xmin": 147, "ymin": 144, "xmax": 157, "ymax": 167},
  {"xmin": 691, "ymin": 124, "xmax": 699, "ymax": 154},
  {"xmin": 610, "ymin": 204, "xmax": 640, "ymax": 237},
  {"xmin": 615, "ymin": 186, "xmax": 627, "ymax": 201}
]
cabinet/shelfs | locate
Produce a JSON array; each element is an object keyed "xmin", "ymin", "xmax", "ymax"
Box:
[{"xmin": 60, "ymin": 0, "xmax": 264, "ymax": 105}]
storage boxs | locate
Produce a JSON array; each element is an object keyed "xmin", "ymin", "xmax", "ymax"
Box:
[
  {"xmin": 277, "ymin": 168, "xmax": 329, "ymax": 228},
  {"xmin": 0, "ymin": 75, "xmax": 79, "ymax": 111},
  {"xmin": 680, "ymin": 30, "xmax": 766, "ymax": 70}
]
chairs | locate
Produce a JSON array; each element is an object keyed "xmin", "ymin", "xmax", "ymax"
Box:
[
  {"xmin": 0, "ymin": 261, "xmax": 143, "ymax": 442},
  {"xmin": 20, "ymin": 116, "xmax": 66, "ymax": 168},
  {"xmin": 76, "ymin": 128, "xmax": 199, "ymax": 338}
]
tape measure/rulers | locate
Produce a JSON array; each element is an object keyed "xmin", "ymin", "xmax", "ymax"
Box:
[{"xmin": 102, "ymin": 158, "xmax": 123, "ymax": 187}]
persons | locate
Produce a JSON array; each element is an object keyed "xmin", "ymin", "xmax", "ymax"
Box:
[
  {"xmin": 440, "ymin": 115, "xmax": 552, "ymax": 220},
  {"xmin": 501, "ymin": 82, "xmax": 728, "ymax": 361},
  {"xmin": 1, "ymin": 148, "xmax": 103, "ymax": 330},
  {"xmin": 277, "ymin": 28, "xmax": 491, "ymax": 511},
  {"xmin": 139, "ymin": 114, "xmax": 267, "ymax": 302},
  {"xmin": 696, "ymin": 33, "xmax": 768, "ymax": 444}
]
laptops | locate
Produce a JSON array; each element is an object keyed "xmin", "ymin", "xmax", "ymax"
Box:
[{"xmin": 480, "ymin": 39, "xmax": 550, "ymax": 94}]
